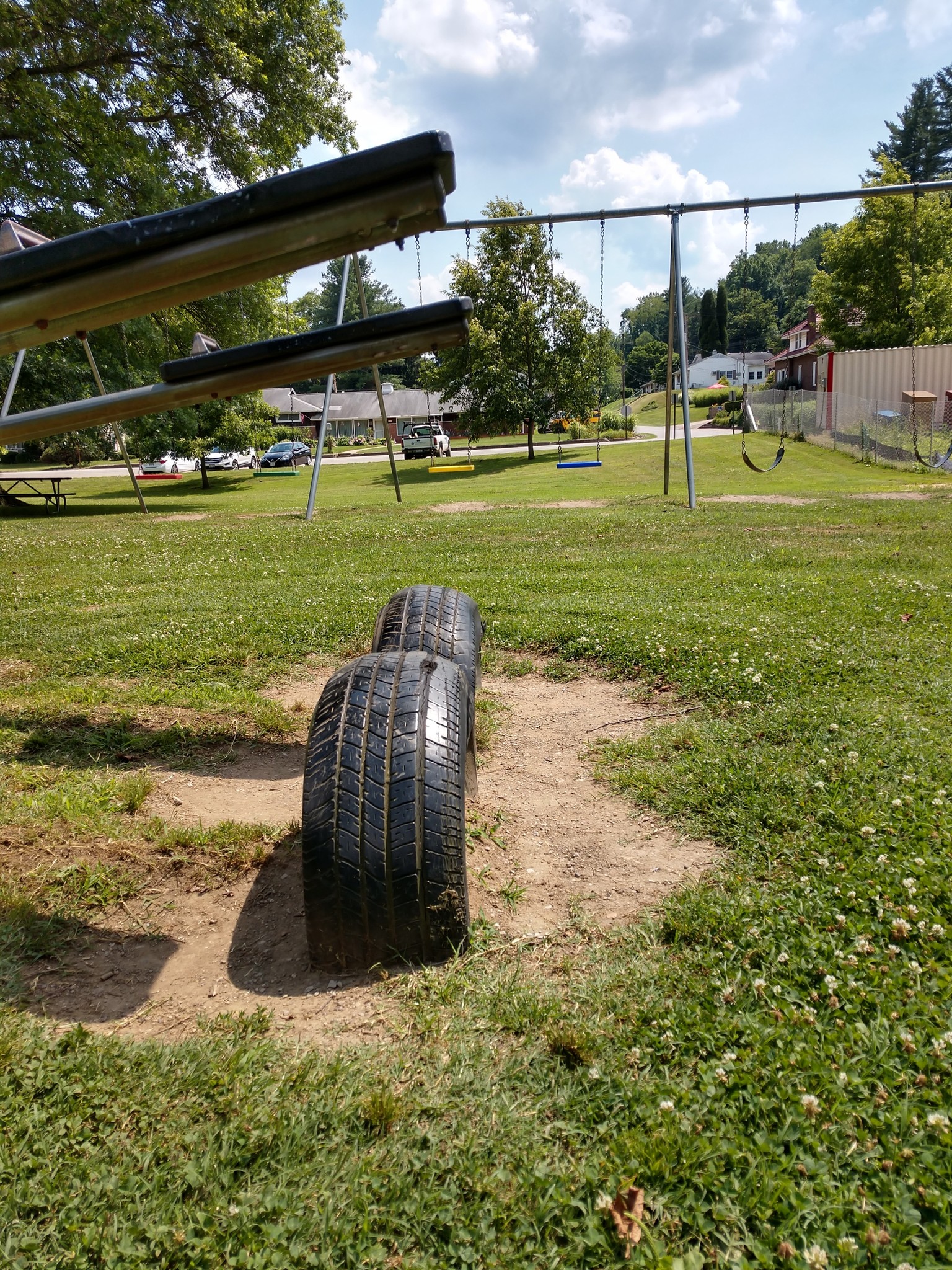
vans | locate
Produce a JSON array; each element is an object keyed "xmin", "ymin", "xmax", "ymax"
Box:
[
  {"xmin": 538, "ymin": 411, "xmax": 576, "ymax": 434},
  {"xmin": 549, "ymin": 410, "xmax": 602, "ymax": 434}
]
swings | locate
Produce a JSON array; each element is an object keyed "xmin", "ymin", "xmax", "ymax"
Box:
[
  {"xmin": 412, "ymin": 228, "xmax": 477, "ymax": 473},
  {"xmin": 237, "ymin": 272, "xmax": 303, "ymax": 480},
  {"xmin": 512, "ymin": 220, "xmax": 607, "ymax": 468},
  {"xmin": 740, "ymin": 200, "xmax": 802, "ymax": 473},
  {"xmin": 120, "ymin": 307, "xmax": 185, "ymax": 481},
  {"xmin": 908, "ymin": 190, "xmax": 951, "ymax": 471}
]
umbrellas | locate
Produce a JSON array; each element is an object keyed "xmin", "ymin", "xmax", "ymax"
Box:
[{"xmin": 707, "ymin": 383, "xmax": 728, "ymax": 390}]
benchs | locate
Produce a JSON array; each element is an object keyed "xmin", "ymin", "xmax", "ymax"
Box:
[{"xmin": 0, "ymin": 493, "xmax": 76, "ymax": 499}]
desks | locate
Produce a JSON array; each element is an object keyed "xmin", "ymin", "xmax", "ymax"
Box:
[{"xmin": 0, "ymin": 478, "xmax": 72, "ymax": 515}]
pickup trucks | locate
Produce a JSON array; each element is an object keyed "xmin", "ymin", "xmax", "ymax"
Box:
[{"xmin": 400, "ymin": 425, "xmax": 451, "ymax": 460}]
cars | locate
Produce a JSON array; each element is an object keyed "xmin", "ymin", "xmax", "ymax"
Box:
[
  {"xmin": 203, "ymin": 444, "xmax": 258, "ymax": 470},
  {"xmin": 139, "ymin": 449, "xmax": 201, "ymax": 474},
  {"xmin": 259, "ymin": 442, "xmax": 315, "ymax": 466}
]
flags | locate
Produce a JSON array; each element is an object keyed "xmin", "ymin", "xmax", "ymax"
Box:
[{"xmin": 300, "ymin": 414, "xmax": 305, "ymax": 424}]
